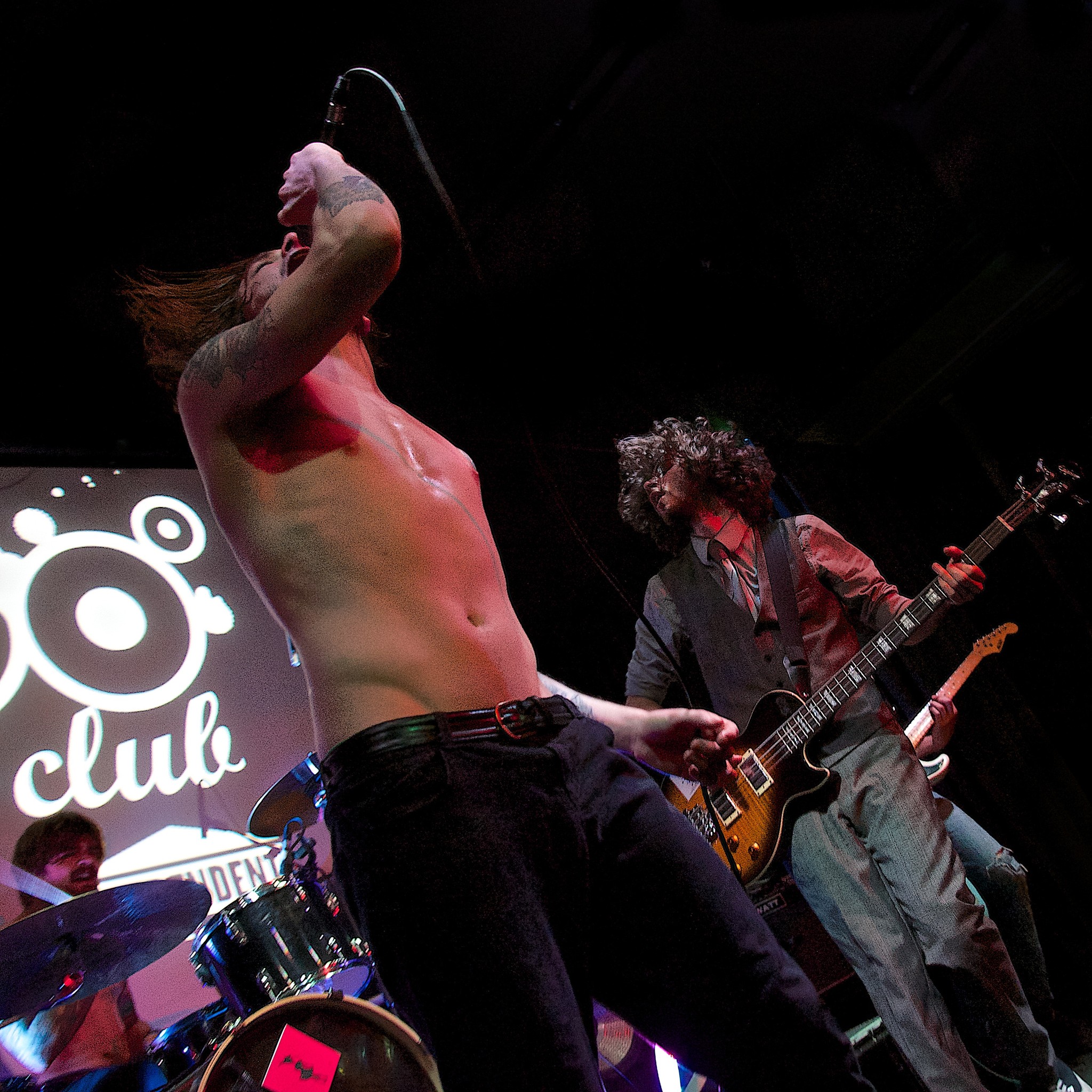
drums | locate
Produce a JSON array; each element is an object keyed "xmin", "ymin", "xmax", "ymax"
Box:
[
  {"xmin": 145, "ymin": 996, "xmax": 241, "ymax": 1083},
  {"xmin": 187, "ymin": 870, "xmax": 376, "ymax": 1023},
  {"xmin": 171, "ymin": 991, "xmax": 444, "ymax": 1092}
]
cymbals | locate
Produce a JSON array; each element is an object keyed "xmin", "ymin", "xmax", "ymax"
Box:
[
  {"xmin": 0, "ymin": 878, "xmax": 213, "ymax": 1022},
  {"xmin": 247, "ymin": 751, "xmax": 322, "ymax": 838}
]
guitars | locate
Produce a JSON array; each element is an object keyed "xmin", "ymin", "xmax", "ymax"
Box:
[
  {"xmin": 903, "ymin": 622, "xmax": 1019, "ymax": 787},
  {"xmin": 661, "ymin": 458, "xmax": 1089, "ymax": 891}
]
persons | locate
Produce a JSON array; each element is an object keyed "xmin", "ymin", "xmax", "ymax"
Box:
[
  {"xmin": 613, "ymin": 415, "xmax": 1092, "ymax": 1092},
  {"xmin": 0, "ymin": 813, "xmax": 160, "ymax": 1084},
  {"xmin": 124, "ymin": 142, "xmax": 875, "ymax": 1092}
]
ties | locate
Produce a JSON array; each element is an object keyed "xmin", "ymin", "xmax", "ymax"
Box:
[{"xmin": 707, "ymin": 539, "xmax": 760, "ymax": 624}]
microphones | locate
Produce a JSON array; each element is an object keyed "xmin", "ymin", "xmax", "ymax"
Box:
[
  {"xmin": 280, "ymin": 827, "xmax": 292, "ymax": 874},
  {"xmin": 294, "ymin": 75, "xmax": 350, "ymax": 247}
]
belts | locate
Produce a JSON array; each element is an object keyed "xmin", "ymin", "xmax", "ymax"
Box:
[{"xmin": 325, "ymin": 693, "xmax": 590, "ymax": 772}]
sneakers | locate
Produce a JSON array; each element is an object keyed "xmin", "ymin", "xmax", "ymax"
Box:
[{"xmin": 1032, "ymin": 1059, "xmax": 1091, "ymax": 1092}]
